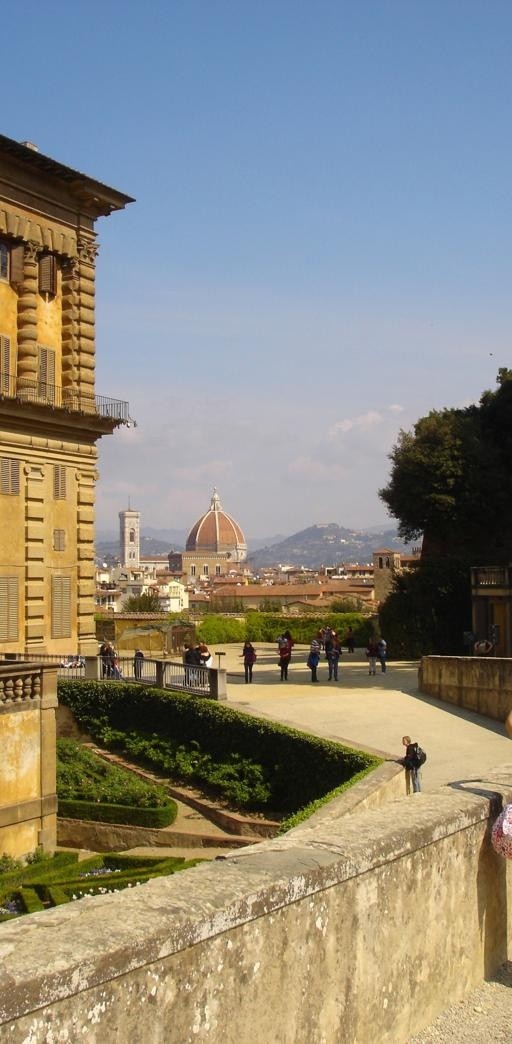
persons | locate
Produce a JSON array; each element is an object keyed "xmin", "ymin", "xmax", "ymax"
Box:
[
  {"xmin": 475, "ymin": 636, "xmax": 494, "ymax": 657},
  {"xmin": 60, "ymin": 639, "xmax": 213, "ymax": 689},
  {"xmin": 402, "ymin": 735, "xmax": 423, "ymax": 793},
  {"xmin": 488, "ymin": 795, "xmax": 512, "ymax": 859},
  {"xmin": 504, "ymin": 710, "xmax": 512, "ymax": 738},
  {"xmin": 239, "ymin": 640, "xmax": 256, "ymax": 683},
  {"xmin": 278, "ymin": 624, "xmax": 388, "ymax": 682}
]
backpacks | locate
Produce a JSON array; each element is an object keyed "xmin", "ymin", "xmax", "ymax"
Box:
[{"xmin": 412, "ymin": 743, "xmax": 426, "ymax": 768}]
